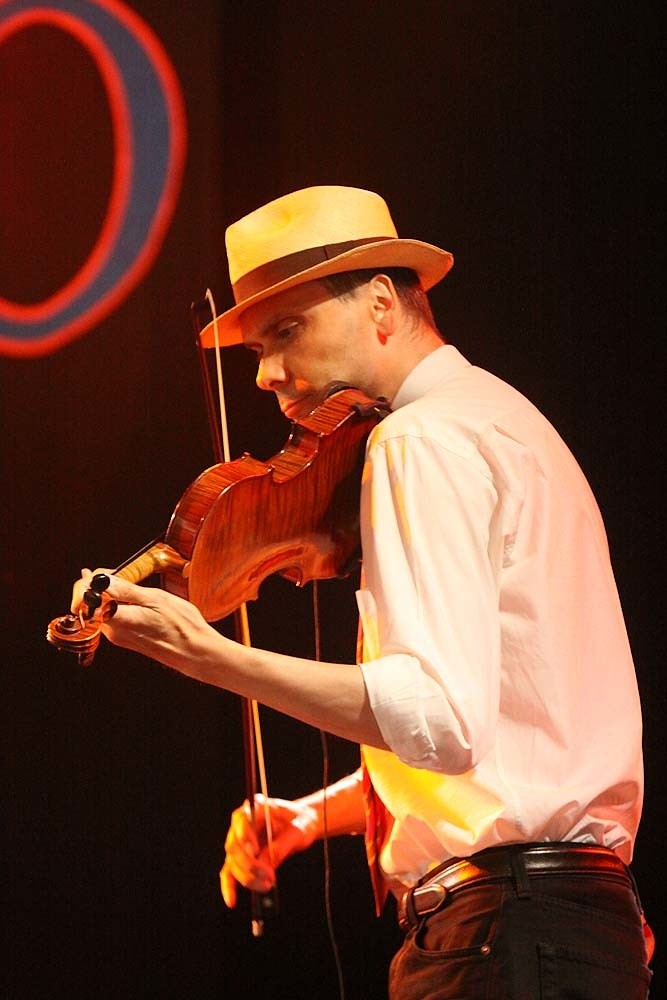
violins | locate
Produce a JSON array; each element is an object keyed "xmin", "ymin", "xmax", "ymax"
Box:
[{"xmin": 45, "ymin": 388, "xmax": 394, "ymax": 671}]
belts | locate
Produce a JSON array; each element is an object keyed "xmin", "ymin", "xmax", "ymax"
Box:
[{"xmin": 397, "ymin": 848, "xmax": 632, "ymax": 928}]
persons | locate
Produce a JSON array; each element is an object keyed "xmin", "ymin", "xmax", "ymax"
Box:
[{"xmin": 72, "ymin": 184, "xmax": 657, "ymax": 1000}]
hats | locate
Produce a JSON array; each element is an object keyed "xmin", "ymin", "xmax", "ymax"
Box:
[{"xmin": 195, "ymin": 184, "xmax": 456, "ymax": 349}]
